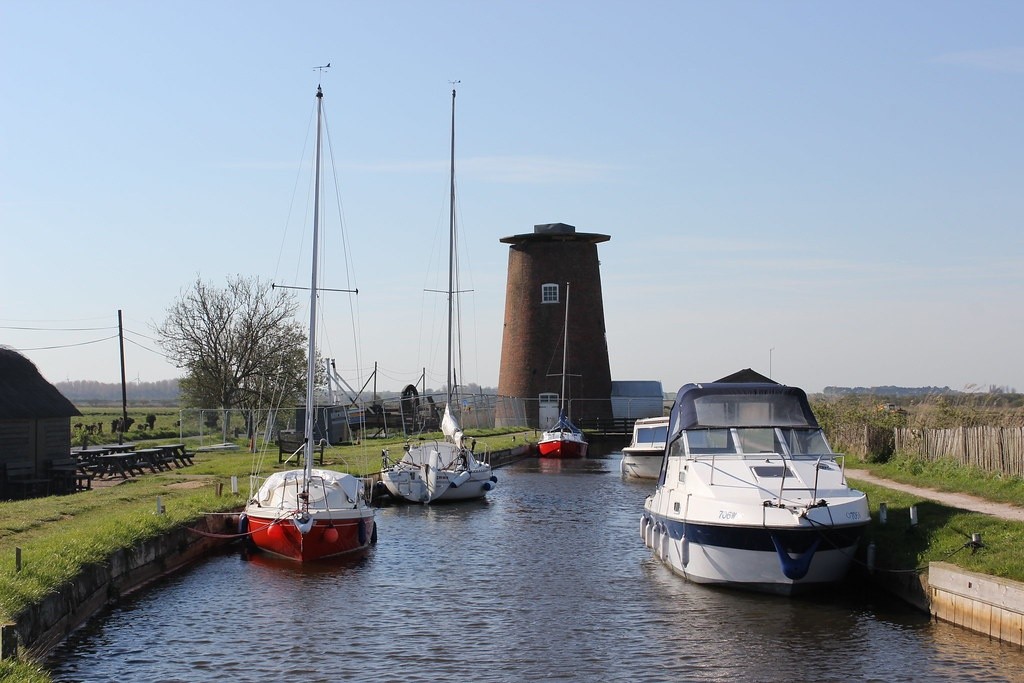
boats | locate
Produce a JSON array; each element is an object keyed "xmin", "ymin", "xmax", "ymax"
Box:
[
  {"xmin": 620, "ymin": 416, "xmax": 709, "ymax": 479},
  {"xmin": 639, "ymin": 368, "xmax": 876, "ymax": 588}
]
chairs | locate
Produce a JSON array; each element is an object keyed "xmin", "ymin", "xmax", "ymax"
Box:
[
  {"xmin": 278, "ymin": 430, "xmax": 324, "ymax": 466},
  {"xmin": 48, "ymin": 457, "xmax": 92, "ymax": 493}
]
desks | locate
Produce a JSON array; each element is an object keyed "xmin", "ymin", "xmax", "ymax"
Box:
[
  {"xmin": 101, "ymin": 445, "xmax": 136, "ymax": 452},
  {"xmin": 98, "ymin": 452, "xmax": 139, "ymax": 479},
  {"xmin": 153, "ymin": 444, "xmax": 193, "ymax": 467},
  {"xmin": 73, "ymin": 449, "xmax": 110, "ymax": 464},
  {"xmin": 132, "ymin": 448, "xmax": 172, "ymax": 472}
]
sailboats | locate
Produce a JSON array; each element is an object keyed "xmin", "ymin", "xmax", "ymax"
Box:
[
  {"xmin": 538, "ymin": 281, "xmax": 589, "ymax": 459},
  {"xmin": 241, "ymin": 63, "xmax": 377, "ymax": 563},
  {"xmin": 379, "ymin": 80, "xmax": 497, "ymax": 504}
]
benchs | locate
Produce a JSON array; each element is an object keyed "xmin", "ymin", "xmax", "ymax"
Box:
[
  {"xmin": 3, "ymin": 457, "xmax": 53, "ymax": 499},
  {"xmin": 69, "ymin": 433, "xmax": 197, "ymax": 479}
]
